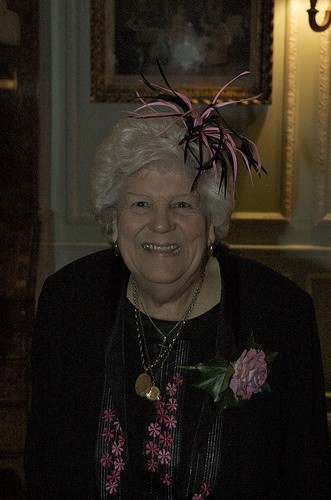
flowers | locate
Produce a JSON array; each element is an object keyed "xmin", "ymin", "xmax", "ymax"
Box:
[{"xmin": 177, "ymin": 329, "xmax": 279, "ymax": 410}]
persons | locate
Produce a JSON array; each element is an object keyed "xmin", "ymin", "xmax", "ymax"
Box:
[{"xmin": 23, "ymin": 114, "xmax": 331, "ymax": 500}]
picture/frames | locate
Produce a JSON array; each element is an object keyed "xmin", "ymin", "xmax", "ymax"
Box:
[{"xmin": 90, "ymin": 0, "xmax": 276, "ymax": 105}]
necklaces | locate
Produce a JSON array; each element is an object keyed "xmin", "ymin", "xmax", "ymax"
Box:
[{"xmin": 129, "ymin": 268, "xmax": 208, "ymax": 401}]
